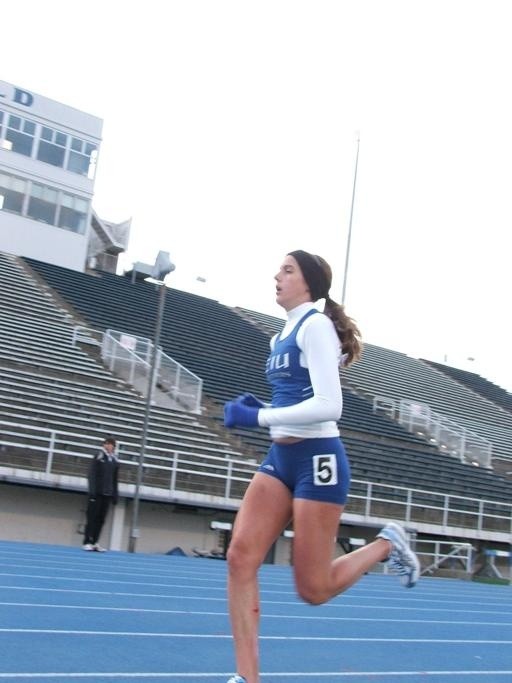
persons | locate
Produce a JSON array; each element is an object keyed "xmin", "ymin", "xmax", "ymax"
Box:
[
  {"xmin": 82, "ymin": 436, "xmax": 122, "ymax": 553},
  {"xmin": 221, "ymin": 248, "xmax": 418, "ymax": 682}
]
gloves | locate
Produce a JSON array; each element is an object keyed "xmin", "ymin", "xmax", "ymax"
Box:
[{"xmin": 221, "ymin": 391, "xmax": 267, "ymax": 430}]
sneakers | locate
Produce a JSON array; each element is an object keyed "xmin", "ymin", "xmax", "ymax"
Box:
[
  {"xmin": 374, "ymin": 520, "xmax": 423, "ymax": 589},
  {"xmin": 82, "ymin": 542, "xmax": 107, "ymax": 553}
]
